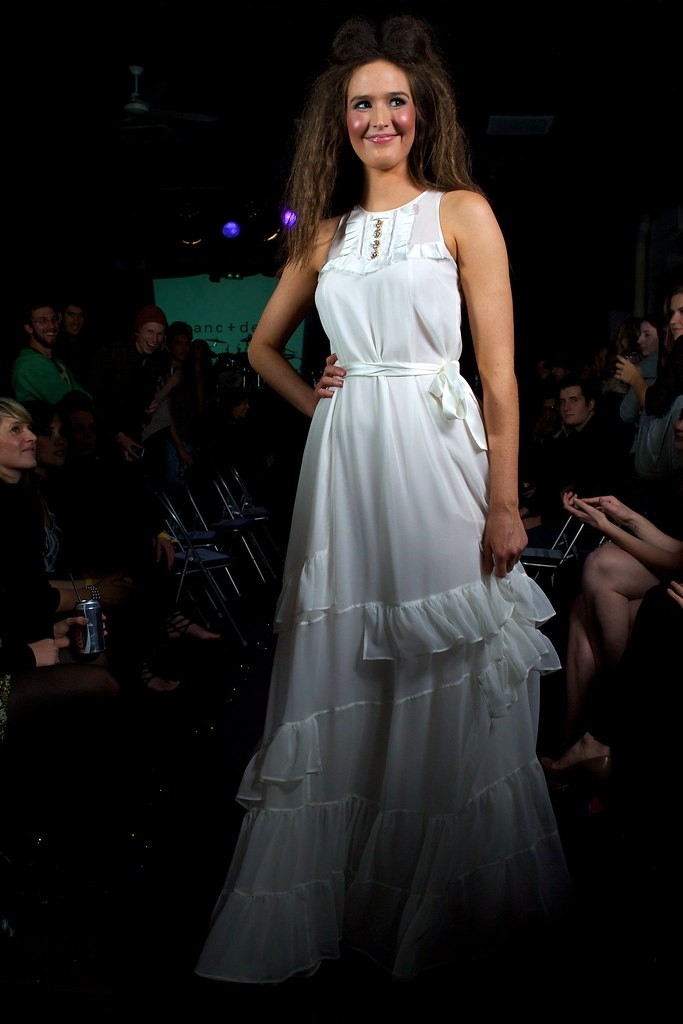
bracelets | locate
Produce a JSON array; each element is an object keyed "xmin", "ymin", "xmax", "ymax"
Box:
[
  {"xmin": 84, "ymin": 585, "xmax": 100, "ymax": 601},
  {"xmin": 158, "ymin": 532, "xmax": 171, "ymax": 542}
]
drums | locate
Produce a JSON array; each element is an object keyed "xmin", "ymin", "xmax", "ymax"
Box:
[{"xmin": 219, "ymin": 352, "xmax": 233, "ymax": 357}]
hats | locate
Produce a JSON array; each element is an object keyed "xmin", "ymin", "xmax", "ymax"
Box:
[
  {"xmin": 219, "ymin": 386, "xmax": 252, "ymax": 412},
  {"xmin": 134, "ymin": 303, "xmax": 169, "ymax": 332}
]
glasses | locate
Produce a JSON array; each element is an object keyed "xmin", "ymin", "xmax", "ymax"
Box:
[
  {"xmin": 542, "ymin": 403, "xmax": 560, "ymax": 414},
  {"xmin": 66, "ymin": 310, "xmax": 88, "ymax": 321},
  {"xmin": 621, "ymin": 352, "xmax": 640, "ymax": 366},
  {"xmin": 31, "ymin": 315, "xmax": 59, "ymax": 327}
]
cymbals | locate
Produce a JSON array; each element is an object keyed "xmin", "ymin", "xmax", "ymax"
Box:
[
  {"xmin": 207, "ymin": 338, "xmax": 226, "ymax": 343},
  {"xmin": 240, "ymin": 336, "xmax": 252, "ymax": 342}
]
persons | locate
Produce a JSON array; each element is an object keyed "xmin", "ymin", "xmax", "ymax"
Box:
[
  {"xmin": 517, "ymin": 279, "xmax": 683, "ymax": 775},
  {"xmin": 194, "ymin": 17, "xmax": 571, "ymax": 984},
  {"xmin": 0, "ymin": 285, "xmax": 305, "ymax": 872}
]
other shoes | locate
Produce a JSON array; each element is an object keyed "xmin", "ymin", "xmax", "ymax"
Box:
[
  {"xmin": 141, "ymin": 667, "xmax": 180, "ymax": 692},
  {"xmin": 162, "ymin": 617, "xmax": 225, "ymax": 643},
  {"xmin": 540, "ymin": 755, "xmax": 612, "ymax": 785}
]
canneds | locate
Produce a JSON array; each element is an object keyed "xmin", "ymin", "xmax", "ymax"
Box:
[
  {"xmin": 619, "ymin": 353, "xmax": 639, "ymax": 387},
  {"xmin": 168, "ymin": 364, "xmax": 177, "ymax": 375},
  {"xmin": 73, "ymin": 599, "xmax": 105, "ymax": 655}
]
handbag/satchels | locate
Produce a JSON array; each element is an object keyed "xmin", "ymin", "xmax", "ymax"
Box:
[{"xmin": 634, "ymin": 395, "xmax": 683, "ymax": 480}]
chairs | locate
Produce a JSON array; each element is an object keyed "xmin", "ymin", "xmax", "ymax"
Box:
[
  {"xmin": 519, "ymin": 515, "xmax": 586, "ymax": 588},
  {"xmin": 159, "ymin": 469, "xmax": 277, "ymax": 648}
]
